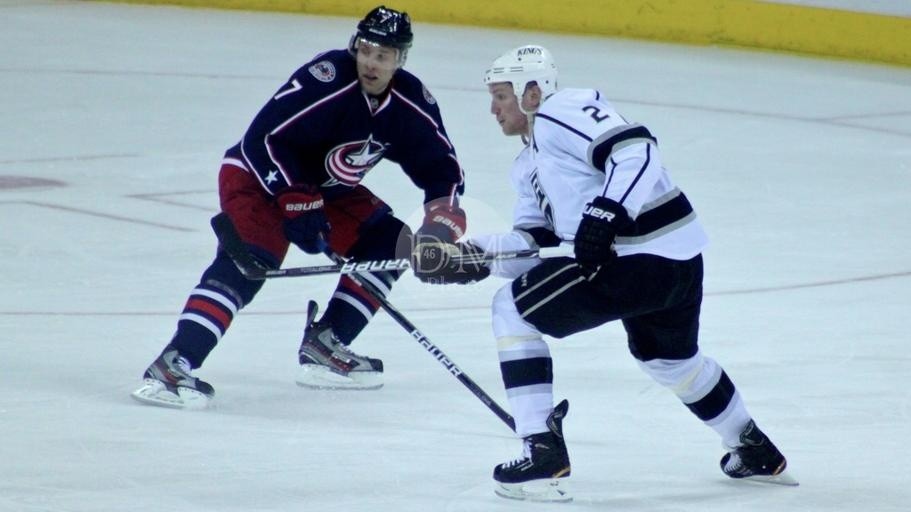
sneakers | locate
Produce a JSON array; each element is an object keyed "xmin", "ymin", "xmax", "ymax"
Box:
[
  {"xmin": 719, "ymin": 443, "xmax": 786, "ymax": 479},
  {"xmin": 299, "ymin": 299, "xmax": 384, "ymax": 375},
  {"xmin": 143, "ymin": 345, "xmax": 216, "ymax": 400},
  {"xmin": 492, "ymin": 432, "xmax": 570, "ymax": 483}
]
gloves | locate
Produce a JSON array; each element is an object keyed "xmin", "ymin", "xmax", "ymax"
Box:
[
  {"xmin": 283, "ymin": 212, "xmax": 333, "ymax": 256},
  {"xmin": 409, "ymin": 219, "xmax": 494, "ymax": 286},
  {"xmin": 572, "ymin": 241, "xmax": 619, "ymax": 269}
]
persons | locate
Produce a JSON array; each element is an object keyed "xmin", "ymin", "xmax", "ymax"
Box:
[
  {"xmin": 141, "ymin": 4, "xmax": 468, "ymax": 397},
  {"xmin": 410, "ymin": 44, "xmax": 789, "ymax": 483}
]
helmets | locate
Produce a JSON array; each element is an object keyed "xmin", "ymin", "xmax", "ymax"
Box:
[
  {"xmin": 481, "ymin": 44, "xmax": 558, "ymax": 115},
  {"xmin": 347, "ymin": 4, "xmax": 415, "ymax": 60}
]
